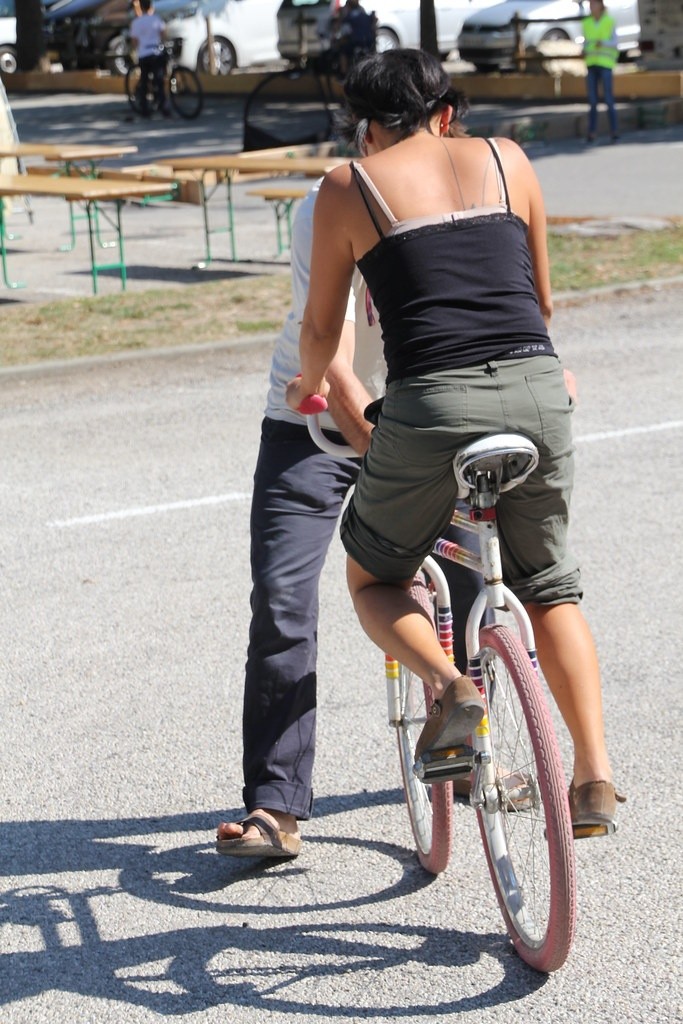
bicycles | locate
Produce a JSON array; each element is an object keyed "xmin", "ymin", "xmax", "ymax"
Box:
[
  {"xmin": 125, "ymin": 38, "xmax": 204, "ymax": 119},
  {"xmin": 288, "ymin": 374, "xmax": 577, "ymax": 971}
]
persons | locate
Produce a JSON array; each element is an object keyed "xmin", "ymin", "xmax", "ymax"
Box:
[
  {"xmin": 580, "ymin": 0, "xmax": 621, "ymax": 142},
  {"xmin": 333, "ymin": 0, "xmax": 376, "ymax": 83},
  {"xmin": 285, "ymin": 51, "xmax": 627, "ymax": 824},
  {"xmin": 216, "ymin": 118, "xmax": 537, "ymax": 856},
  {"xmin": 129, "ymin": 0, "xmax": 172, "ymax": 116}
]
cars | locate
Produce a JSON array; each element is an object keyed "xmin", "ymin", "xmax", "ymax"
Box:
[
  {"xmin": 0, "ymin": 0, "xmax": 58, "ymax": 74},
  {"xmin": 457, "ymin": 0, "xmax": 640, "ymax": 72},
  {"xmin": 106, "ymin": 1, "xmax": 285, "ymax": 77},
  {"xmin": 42, "ymin": 0, "xmax": 189, "ymax": 66},
  {"xmin": 277, "ymin": 0, "xmax": 504, "ymax": 62}
]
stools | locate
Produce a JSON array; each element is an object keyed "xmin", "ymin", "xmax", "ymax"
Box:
[{"xmin": 245, "ymin": 188, "xmax": 308, "ymax": 255}]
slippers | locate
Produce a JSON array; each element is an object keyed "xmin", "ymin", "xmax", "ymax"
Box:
[
  {"xmin": 454, "ymin": 770, "xmax": 536, "ymax": 810},
  {"xmin": 216, "ymin": 807, "xmax": 302, "ymax": 858}
]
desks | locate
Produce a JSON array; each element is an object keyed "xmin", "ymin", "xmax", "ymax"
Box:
[
  {"xmin": 0, "ymin": 174, "xmax": 173, "ymax": 296},
  {"xmin": 153, "ymin": 154, "xmax": 361, "ymax": 270},
  {"xmin": 0, "ymin": 141, "xmax": 139, "ymax": 253}
]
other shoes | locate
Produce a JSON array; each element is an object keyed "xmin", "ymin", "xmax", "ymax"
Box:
[
  {"xmin": 570, "ymin": 776, "xmax": 627, "ymax": 828},
  {"xmin": 611, "ymin": 134, "xmax": 619, "ymax": 141},
  {"xmin": 585, "ymin": 135, "xmax": 593, "ymax": 144},
  {"xmin": 415, "ymin": 676, "xmax": 486, "ymax": 753}
]
